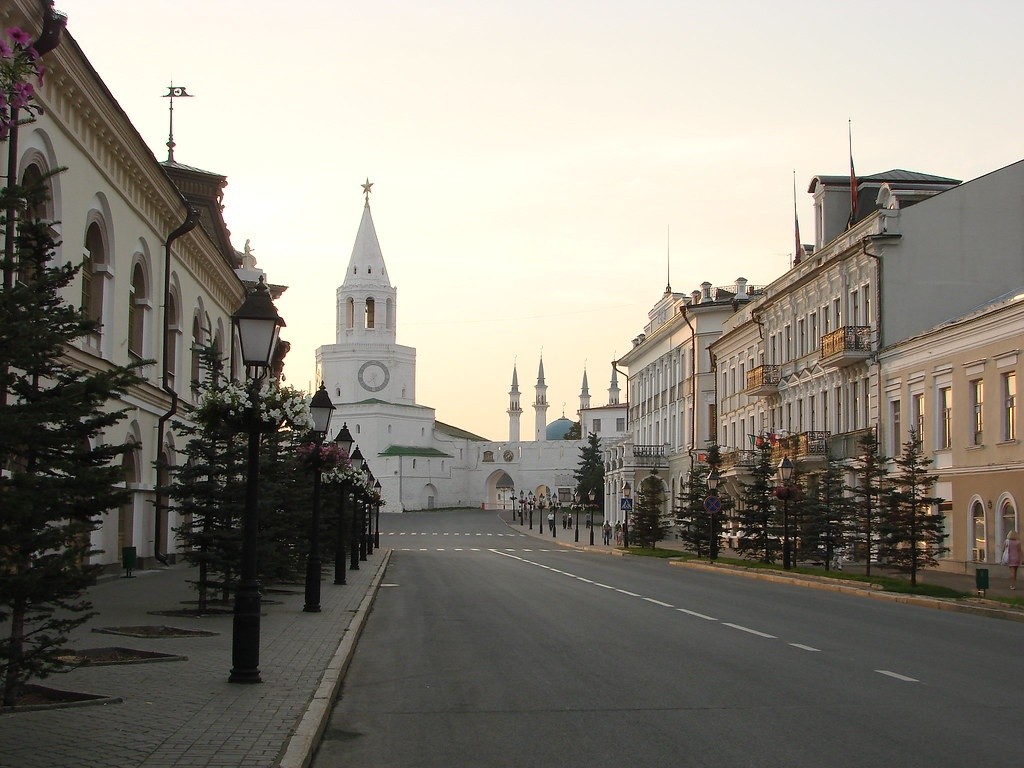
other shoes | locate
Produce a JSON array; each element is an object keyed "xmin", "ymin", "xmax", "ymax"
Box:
[{"xmin": 1010, "ymin": 585, "xmax": 1016, "ymax": 590}]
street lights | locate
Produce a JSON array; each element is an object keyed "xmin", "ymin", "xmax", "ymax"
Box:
[
  {"xmin": 778, "ymin": 453, "xmax": 794, "ymax": 570},
  {"xmin": 552, "ymin": 492, "xmax": 558, "ymax": 537},
  {"xmin": 539, "ymin": 493, "xmax": 545, "ymax": 534},
  {"xmin": 303, "ymin": 379, "xmax": 337, "ymax": 611},
  {"xmin": 366, "ymin": 473, "xmax": 376, "ymax": 555},
  {"xmin": 574, "ymin": 490, "xmax": 582, "ymax": 542},
  {"xmin": 520, "ymin": 489, "xmax": 525, "ymax": 526},
  {"xmin": 359, "ymin": 461, "xmax": 370, "ymax": 561},
  {"xmin": 706, "ymin": 468, "xmax": 721, "ymax": 559},
  {"xmin": 588, "ymin": 488, "xmax": 597, "ymax": 545},
  {"xmin": 350, "ymin": 444, "xmax": 365, "ymax": 570},
  {"xmin": 528, "ymin": 491, "xmax": 534, "ymax": 529},
  {"xmin": 373, "ymin": 479, "xmax": 382, "ymax": 549},
  {"xmin": 622, "ymin": 481, "xmax": 632, "ymax": 548},
  {"xmin": 228, "ymin": 273, "xmax": 286, "ymax": 684},
  {"xmin": 333, "ymin": 420, "xmax": 355, "ymax": 585},
  {"xmin": 511, "ymin": 487, "xmax": 515, "ymax": 521}
]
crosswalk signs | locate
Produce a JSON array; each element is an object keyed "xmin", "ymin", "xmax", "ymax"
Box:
[{"xmin": 620, "ymin": 497, "xmax": 633, "ymax": 511}]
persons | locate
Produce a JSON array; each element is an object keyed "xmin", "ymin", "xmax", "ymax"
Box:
[
  {"xmin": 832, "ymin": 532, "xmax": 845, "ymax": 571},
  {"xmin": 547, "ymin": 508, "xmax": 554, "ymax": 531},
  {"xmin": 603, "ymin": 519, "xmax": 636, "ymax": 546},
  {"xmin": 518, "ymin": 504, "xmax": 528, "ymax": 519},
  {"xmin": 567, "ymin": 513, "xmax": 572, "ymax": 529},
  {"xmin": 1001, "ymin": 529, "xmax": 1022, "ymax": 590},
  {"xmin": 586, "ymin": 511, "xmax": 591, "ymax": 529},
  {"xmin": 563, "ymin": 511, "xmax": 568, "ymax": 528}
]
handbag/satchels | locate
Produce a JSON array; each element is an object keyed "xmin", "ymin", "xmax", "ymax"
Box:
[{"xmin": 1002, "ymin": 540, "xmax": 1010, "ymax": 565}]
